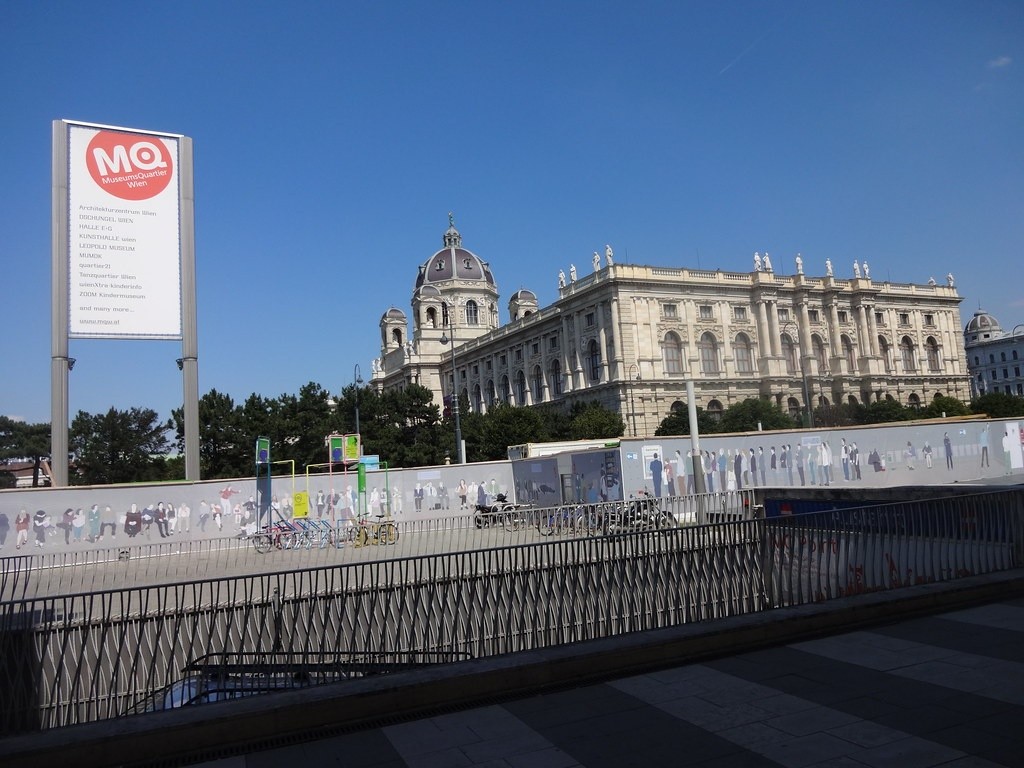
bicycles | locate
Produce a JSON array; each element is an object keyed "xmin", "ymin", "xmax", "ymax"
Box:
[
  {"xmin": 252, "ymin": 512, "xmax": 400, "ymax": 554},
  {"xmin": 504, "ymin": 496, "xmax": 628, "ymax": 537}
]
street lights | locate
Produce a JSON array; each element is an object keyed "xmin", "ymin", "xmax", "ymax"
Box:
[
  {"xmin": 440, "ymin": 313, "xmax": 462, "ymax": 465},
  {"xmin": 782, "ymin": 320, "xmax": 813, "ymax": 426},
  {"xmin": 353, "ymin": 362, "xmax": 364, "ymax": 434}
]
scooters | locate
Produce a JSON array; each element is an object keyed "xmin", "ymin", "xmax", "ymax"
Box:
[{"xmin": 472, "ymin": 489, "xmax": 519, "ymax": 529}]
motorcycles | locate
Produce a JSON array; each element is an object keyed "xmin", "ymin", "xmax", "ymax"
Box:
[{"xmin": 603, "ymin": 492, "xmax": 679, "ymax": 543}]
